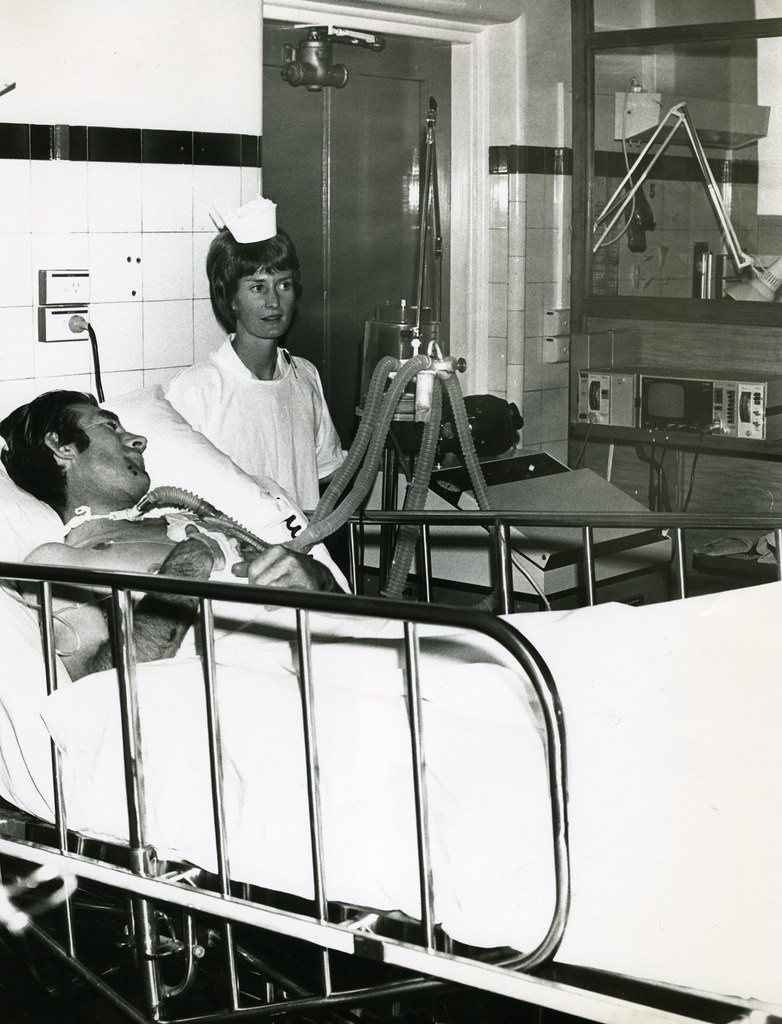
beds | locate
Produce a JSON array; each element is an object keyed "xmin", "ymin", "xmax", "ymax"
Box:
[{"xmin": 0, "ymin": 383, "xmax": 782, "ymax": 1024}]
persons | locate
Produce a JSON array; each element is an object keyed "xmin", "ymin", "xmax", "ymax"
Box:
[
  {"xmin": 0, "ymin": 390, "xmax": 349, "ymax": 682},
  {"xmin": 162, "ymin": 195, "xmax": 349, "ymax": 512}
]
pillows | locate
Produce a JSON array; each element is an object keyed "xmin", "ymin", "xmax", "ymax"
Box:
[{"xmin": 0, "ymin": 384, "xmax": 281, "ymax": 616}]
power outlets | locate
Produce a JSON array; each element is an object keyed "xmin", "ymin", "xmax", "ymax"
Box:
[
  {"xmin": 39, "ymin": 269, "xmax": 90, "ymax": 306},
  {"xmin": 38, "ymin": 305, "xmax": 89, "ymax": 343}
]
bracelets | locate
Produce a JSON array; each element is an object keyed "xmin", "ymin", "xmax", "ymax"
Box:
[{"xmin": 185, "ymin": 532, "xmax": 223, "ymax": 572}]
